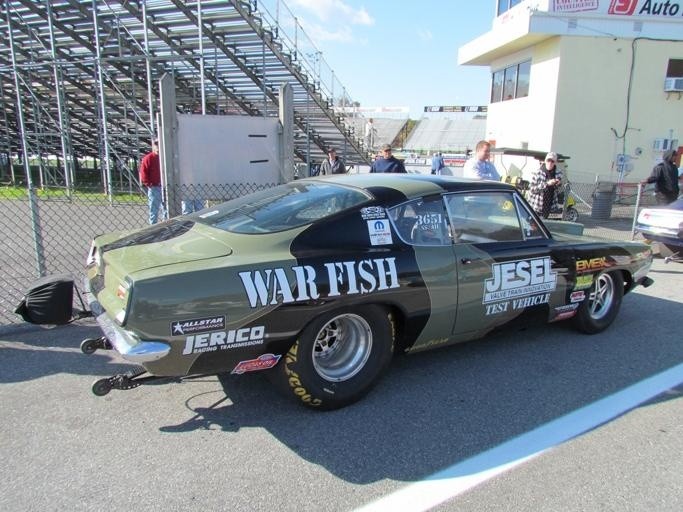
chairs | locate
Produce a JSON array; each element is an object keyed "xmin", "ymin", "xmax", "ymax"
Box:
[{"xmin": 411, "ymin": 199, "xmax": 450, "ymax": 243}]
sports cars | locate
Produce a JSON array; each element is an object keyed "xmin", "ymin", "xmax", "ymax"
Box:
[{"xmin": 80, "ymin": 173, "xmax": 653, "ymax": 409}]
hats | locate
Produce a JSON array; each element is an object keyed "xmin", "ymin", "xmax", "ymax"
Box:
[
  {"xmin": 328, "ymin": 148, "xmax": 334, "ymax": 153},
  {"xmin": 383, "ymin": 144, "xmax": 392, "ymax": 151},
  {"xmin": 545, "ymin": 152, "xmax": 557, "ymax": 162}
]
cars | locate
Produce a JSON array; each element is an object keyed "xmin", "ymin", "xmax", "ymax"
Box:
[{"xmin": 635, "ymin": 193, "xmax": 683, "ymax": 248}]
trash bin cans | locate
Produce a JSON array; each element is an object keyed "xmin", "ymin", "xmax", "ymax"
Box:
[{"xmin": 591, "ymin": 192, "xmax": 616, "ymax": 219}]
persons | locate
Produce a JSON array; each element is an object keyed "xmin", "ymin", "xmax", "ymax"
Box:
[
  {"xmin": 639, "ymin": 147, "xmax": 680, "ymax": 208},
  {"xmin": 429, "ymin": 152, "xmax": 437, "ymax": 174},
  {"xmin": 319, "ymin": 147, "xmax": 347, "ymax": 176},
  {"xmin": 362, "ymin": 116, "xmax": 377, "ymax": 151},
  {"xmin": 461, "ymin": 139, "xmax": 502, "ymax": 181},
  {"xmin": 138, "ymin": 139, "xmax": 167, "ymax": 225},
  {"xmin": 525, "ymin": 150, "xmax": 563, "ymax": 219},
  {"xmin": 367, "ymin": 143, "xmax": 408, "ymax": 173},
  {"xmin": 180, "ymin": 199, "xmax": 203, "ymax": 216},
  {"xmin": 434, "ymin": 151, "xmax": 444, "ymax": 175}
]
